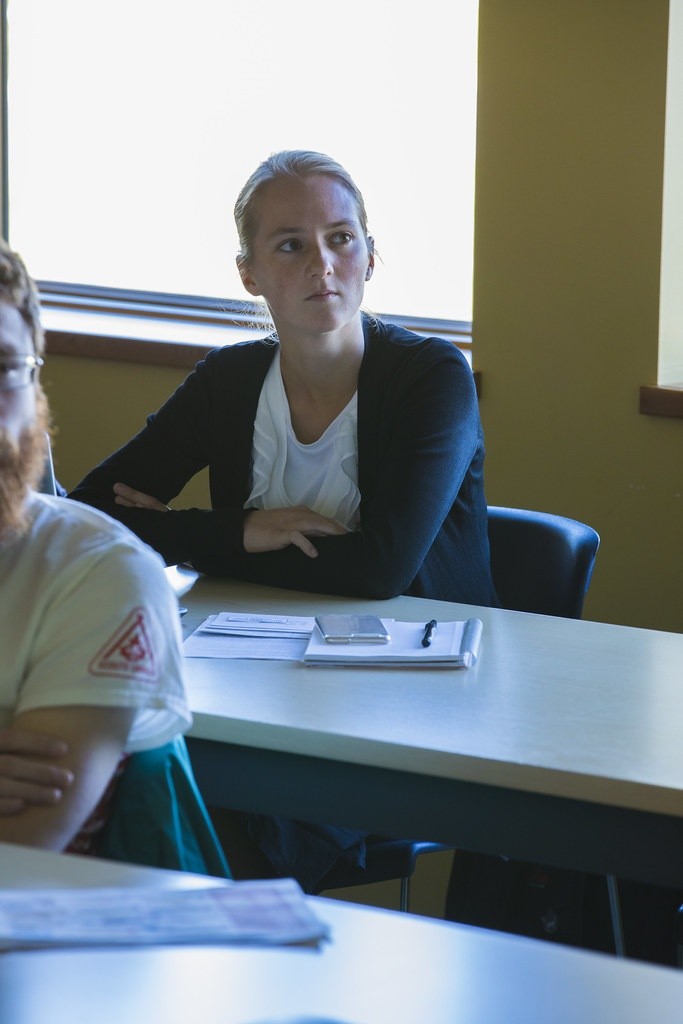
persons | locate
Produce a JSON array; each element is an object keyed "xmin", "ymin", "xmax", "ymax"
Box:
[
  {"xmin": 67, "ymin": 150, "xmax": 502, "ymax": 608},
  {"xmin": 1, "ymin": 242, "xmax": 193, "ymax": 852}
]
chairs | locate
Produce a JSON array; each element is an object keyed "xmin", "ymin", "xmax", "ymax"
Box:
[{"xmin": 313, "ymin": 505, "xmax": 627, "ymax": 957}]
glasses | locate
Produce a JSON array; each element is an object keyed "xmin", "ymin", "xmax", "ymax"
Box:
[{"xmin": 0, "ymin": 353, "xmax": 43, "ymax": 392}]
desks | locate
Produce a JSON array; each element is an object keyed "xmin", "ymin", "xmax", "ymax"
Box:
[{"xmin": 0, "ymin": 563, "xmax": 683, "ymax": 1024}]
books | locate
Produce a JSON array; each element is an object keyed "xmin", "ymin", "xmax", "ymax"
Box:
[{"xmin": 303, "ymin": 614, "xmax": 483, "ymax": 669}]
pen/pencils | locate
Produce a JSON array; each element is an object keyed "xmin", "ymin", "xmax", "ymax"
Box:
[{"xmin": 421, "ymin": 619, "xmax": 437, "ymax": 648}]
